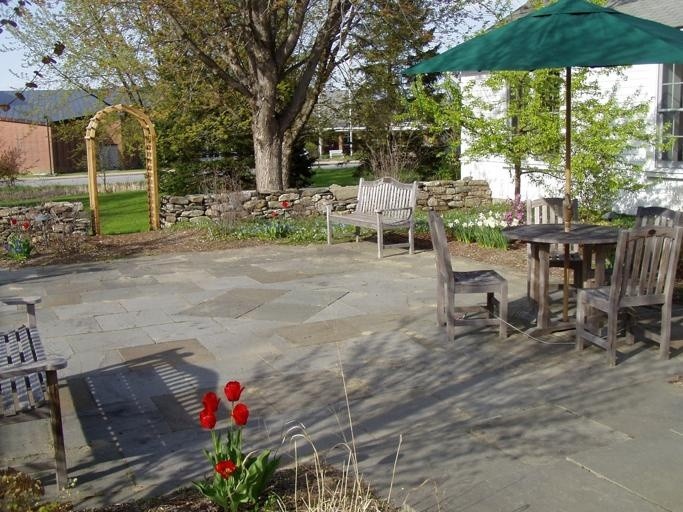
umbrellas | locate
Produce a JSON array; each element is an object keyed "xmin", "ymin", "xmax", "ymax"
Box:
[{"xmin": 401, "ymin": 0, "xmax": 683, "ymax": 322}]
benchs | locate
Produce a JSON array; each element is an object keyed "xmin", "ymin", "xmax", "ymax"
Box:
[
  {"xmin": 0, "ymin": 294, "xmax": 74, "ymax": 493},
  {"xmin": 323, "ymin": 178, "xmax": 417, "ymax": 258}
]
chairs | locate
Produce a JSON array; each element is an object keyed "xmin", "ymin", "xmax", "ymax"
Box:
[
  {"xmin": 586, "ymin": 204, "xmax": 682, "ymax": 285},
  {"xmin": 524, "ymin": 195, "xmax": 581, "ymax": 300},
  {"xmin": 420, "ymin": 207, "xmax": 509, "ymax": 342},
  {"xmin": 574, "ymin": 224, "xmax": 681, "ymax": 368}
]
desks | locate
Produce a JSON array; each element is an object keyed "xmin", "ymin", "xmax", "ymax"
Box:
[{"xmin": 500, "ymin": 223, "xmax": 621, "ymax": 345}]
